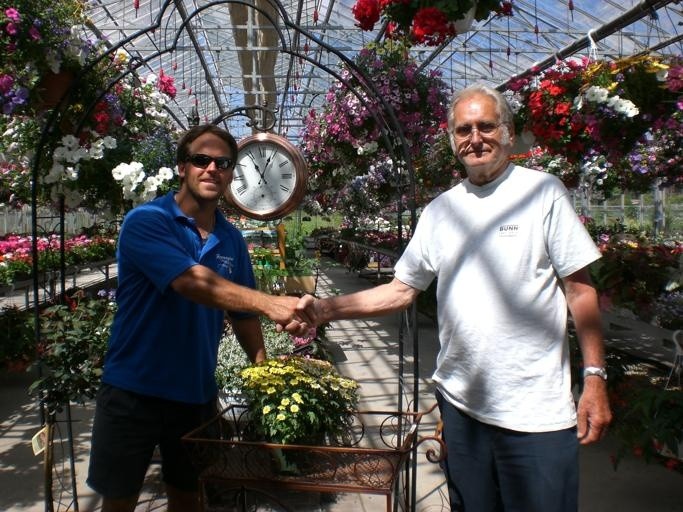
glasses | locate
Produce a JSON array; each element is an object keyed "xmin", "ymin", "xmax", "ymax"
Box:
[
  {"xmin": 449, "ymin": 121, "xmax": 504, "ymax": 142},
  {"xmin": 183, "ymin": 153, "xmax": 234, "ymax": 174}
]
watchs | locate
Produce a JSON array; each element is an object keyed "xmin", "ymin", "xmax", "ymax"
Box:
[{"xmin": 583, "ymin": 366, "xmax": 608, "ymax": 381}]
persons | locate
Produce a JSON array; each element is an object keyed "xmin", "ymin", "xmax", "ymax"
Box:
[
  {"xmin": 274, "ymin": 83, "xmax": 612, "ymax": 512},
  {"xmin": 86, "ymin": 124, "xmax": 318, "ymax": 512}
]
